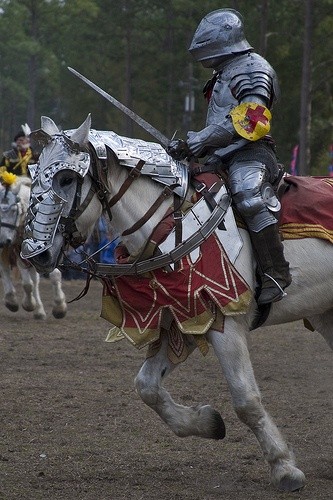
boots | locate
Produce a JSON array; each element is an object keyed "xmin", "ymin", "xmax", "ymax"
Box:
[{"xmin": 249, "ymin": 224, "xmax": 292, "ymax": 302}]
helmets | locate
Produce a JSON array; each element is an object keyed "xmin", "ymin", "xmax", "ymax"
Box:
[{"xmin": 188, "ymin": 7, "xmax": 253, "ymax": 68}]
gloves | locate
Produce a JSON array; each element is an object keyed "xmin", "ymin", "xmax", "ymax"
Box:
[{"xmin": 185, "ymin": 123, "xmax": 234, "ymax": 158}]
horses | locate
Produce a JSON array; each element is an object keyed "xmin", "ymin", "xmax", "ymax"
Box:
[
  {"xmin": 19, "ymin": 112, "xmax": 333, "ymax": 490},
  {"xmin": 2, "ymin": 177, "xmax": 68, "ymax": 318}
]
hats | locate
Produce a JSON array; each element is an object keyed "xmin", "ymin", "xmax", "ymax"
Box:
[{"xmin": 15, "ymin": 131, "xmax": 25, "ymax": 141}]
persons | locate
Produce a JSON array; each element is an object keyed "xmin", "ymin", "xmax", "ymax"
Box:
[
  {"xmin": 162, "ymin": 8, "xmax": 294, "ymax": 305},
  {"xmin": 0, "ymin": 131, "xmax": 41, "ymax": 180}
]
